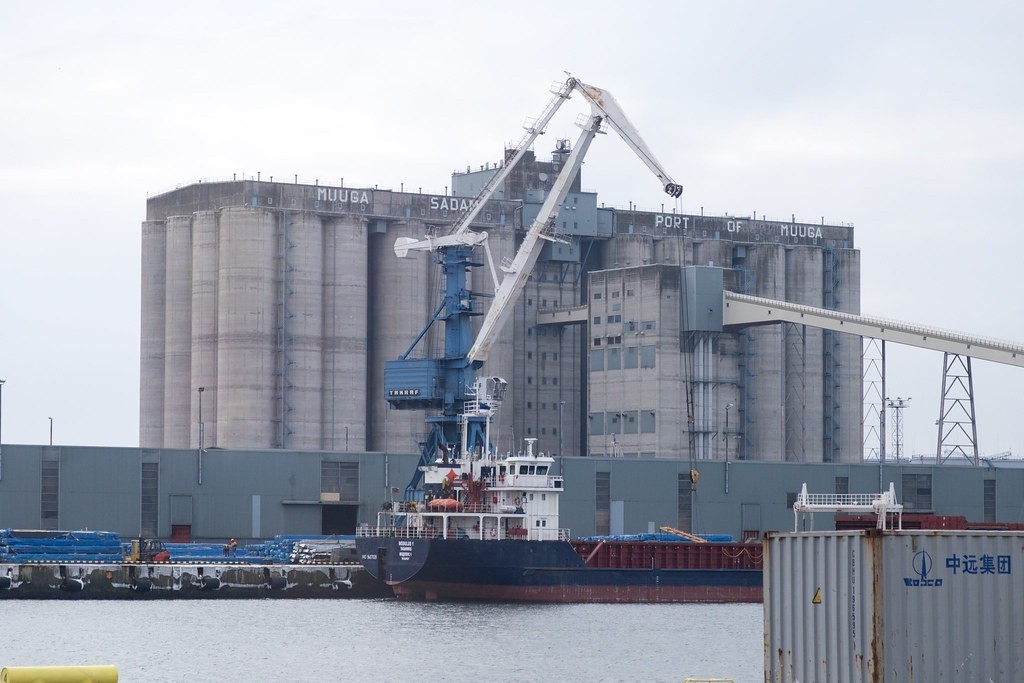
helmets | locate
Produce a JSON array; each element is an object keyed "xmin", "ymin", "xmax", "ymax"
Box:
[{"xmin": 231, "ymin": 539, "xmax": 234, "ymax": 541}]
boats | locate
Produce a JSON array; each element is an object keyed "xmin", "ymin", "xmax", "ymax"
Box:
[{"xmin": 353, "ymin": 68, "xmax": 1024, "ymax": 603}]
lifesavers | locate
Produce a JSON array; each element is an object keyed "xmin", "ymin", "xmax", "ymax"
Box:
[
  {"xmin": 489, "ymin": 528, "xmax": 498, "ymax": 537},
  {"xmin": 514, "ymin": 496, "xmax": 520, "ymax": 505}
]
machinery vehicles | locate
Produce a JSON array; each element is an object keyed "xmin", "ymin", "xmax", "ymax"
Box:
[{"xmin": 126, "ymin": 539, "xmax": 170, "ymax": 561}]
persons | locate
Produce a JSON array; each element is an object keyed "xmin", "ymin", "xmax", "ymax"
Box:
[{"xmin": 230, "ymin": 538, "xmax": 238, "ymax": 557}]
